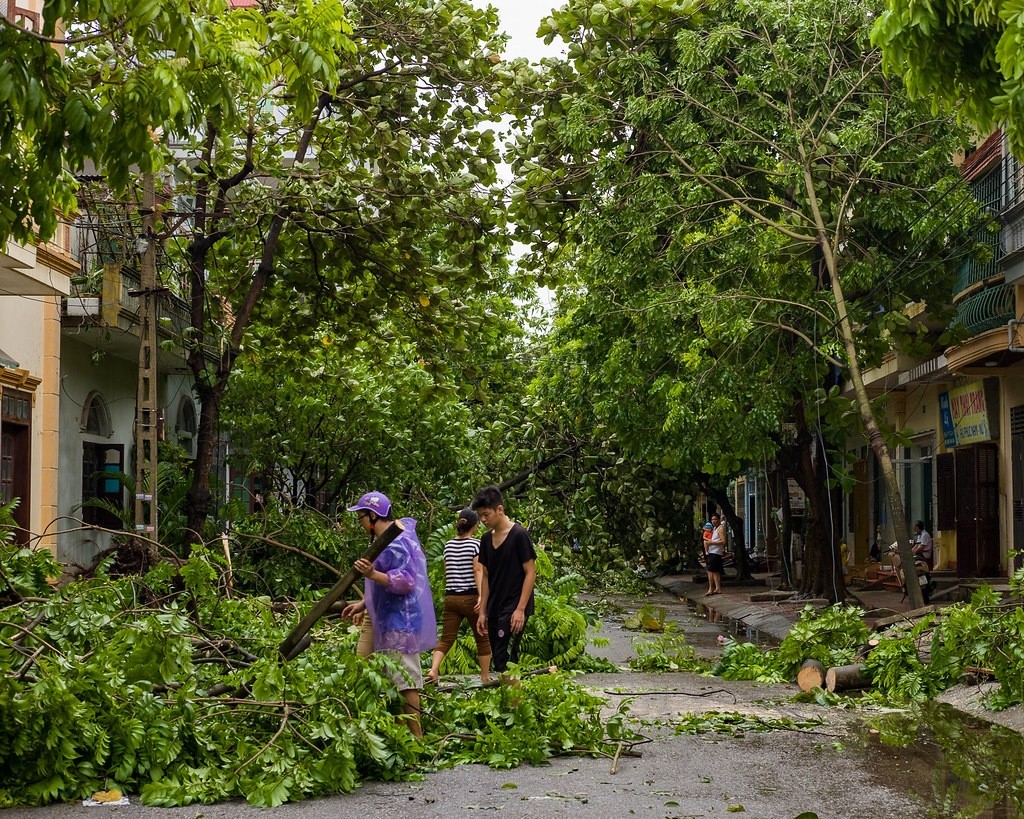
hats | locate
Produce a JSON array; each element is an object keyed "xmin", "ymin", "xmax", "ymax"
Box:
[{"xmin": 703, "ymin": 522, "xmax": 713, "ymax": 530}]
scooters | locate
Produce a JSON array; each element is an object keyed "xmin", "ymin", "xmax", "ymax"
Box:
[{"xmin": 875, "ymin": 531, "xmax": 937, "ymax": 606}]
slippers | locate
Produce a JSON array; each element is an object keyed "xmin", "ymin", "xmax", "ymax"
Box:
[
  {"xmin": 713, "ymin": 592, "xmax": 722, "ymax": 594},
  {"xmin": 705, "ymin": 593, "xmax": 714, "ymax": 596},
  {"xmin": 424, "ymin": 678, "xmax": 439, "ymax": 687}
]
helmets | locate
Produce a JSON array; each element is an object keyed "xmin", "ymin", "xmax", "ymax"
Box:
[{"xmin": 347, "ymin": 490, "xmax": 391, "ymax": 517}]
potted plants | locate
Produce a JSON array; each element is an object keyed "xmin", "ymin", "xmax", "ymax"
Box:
[{"xmin": 80, "ymin": 251, "xmax": 103, "ymax": 301}]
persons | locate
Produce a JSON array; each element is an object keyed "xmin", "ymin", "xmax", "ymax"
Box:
[
  {"xmin": 470, "ymin": 486, "xmax": 538, "ymax": 705},
  {"xmin": 425, "ymin": 511, "xmax": 495, "ymax": 683},
  {"xmin": 841, "ymin": 520, "xmax": 933, "ymax": 590},
  {"xmin": 346, "ymin": 491, "xmax": 438, "ymax": 744},
  {"xmin": 702, "ymin": 514, "xmax": 728, "ymax": 597}
]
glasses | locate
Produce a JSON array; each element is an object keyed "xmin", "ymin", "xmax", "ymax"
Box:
[{"xmin": 358, "ymin": 514, "xmax": 368, "ymax": 523}]
